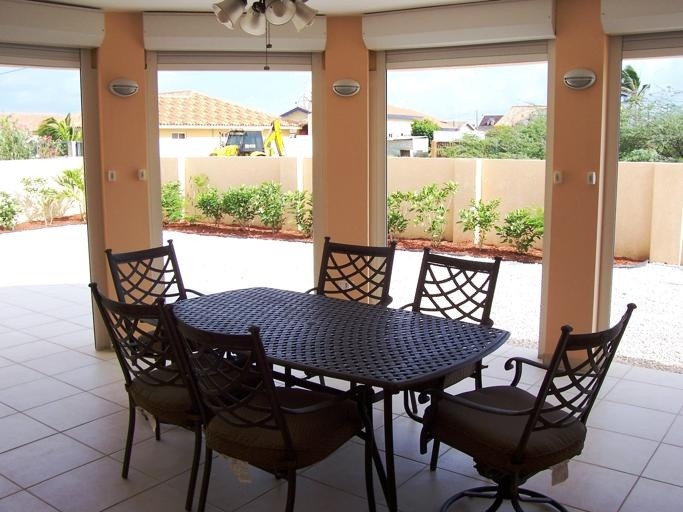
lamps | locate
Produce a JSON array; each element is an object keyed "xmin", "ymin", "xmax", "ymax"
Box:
[
  {"xmin": 108, "ymin": 77, "xmax": 139, "ymax": 98},
  {"xmin": 211, "ymin": 0, "xmax": 318, "ymax": 70},
  {"xmin": 332, "ymin": 79, "xmax": 359, "ymax": 97},
  {"xmin": 563, "ymin": 68, "xmax": 595, "ymax": 91}
]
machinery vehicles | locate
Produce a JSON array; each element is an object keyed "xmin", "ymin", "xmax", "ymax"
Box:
[{"xmin": 209, "ymin": 119, "xmax": 287, "ymax": 156}]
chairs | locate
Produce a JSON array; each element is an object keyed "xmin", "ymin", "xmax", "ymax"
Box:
[
  {"xmin": 364, "ymin": 247, "xmax": 502, "ymax": 422},
  {"xmin": 164, "ymin": 304, "xmax": 377, "ymax": 512},
  {"xmin": 418, "ymin": 302, "xmax": 636, "ymax": 512},
  {"xmin": 104, "ymin": 240, "xmax": 207, "ymax": 368},
  {"xmin": 88, "ymin": 282, "xmax": 266, "ymax": 512},
  {"xmin": 302, "ymin": 236, "xmax": 396, "ymax": 391}
]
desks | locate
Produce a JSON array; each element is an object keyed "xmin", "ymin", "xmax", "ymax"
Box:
[{"xmin": 171, "ymin": 287, "xmax": 511, "ymax": 512}]
